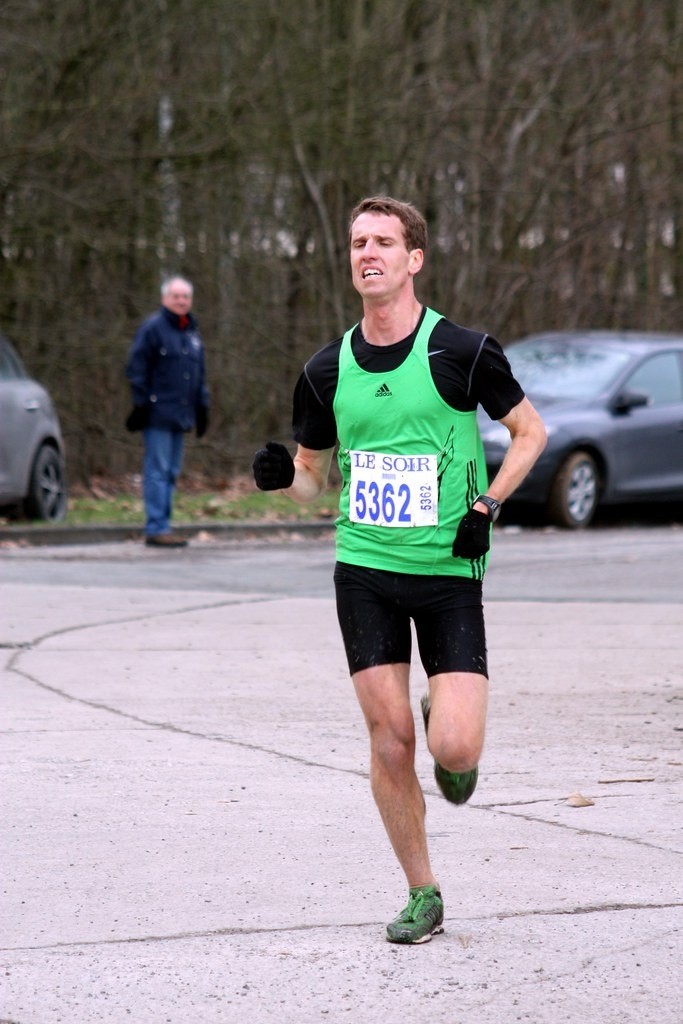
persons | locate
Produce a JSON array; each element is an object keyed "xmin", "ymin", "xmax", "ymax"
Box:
[
  {"xmin": 252, "ymin": 196, "xmax": 548, "ymax": 943},
  {"xmin": 120, "ymin": 273, "xmax": 213, "ymax": 546}
]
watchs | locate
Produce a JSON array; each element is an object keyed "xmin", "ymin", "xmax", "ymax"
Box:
[{"xmin": 474, "ymin": 494, "xmax": 501, "ymax": 521}]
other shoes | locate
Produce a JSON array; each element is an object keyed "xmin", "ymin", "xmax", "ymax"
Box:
[{"xmin": 145, "ymin": 535, "xmax": 187, "ymax": 548}]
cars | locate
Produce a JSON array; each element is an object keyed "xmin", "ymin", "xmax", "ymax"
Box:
[
  {"xmin": 472, "ymin": 329, "xmax": 683, "ymax": 533},
  {"xmin": 0, "ymin": 335, "xmax": 70, "ymax": 525}
]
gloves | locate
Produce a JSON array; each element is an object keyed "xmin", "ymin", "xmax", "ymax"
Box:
[
  {"xmin": 195, "ymin": 419, "xmax": 208, "ymax": 439},
  {"xmin": 252, "ymin": 441, "xmax": 295, "ymax": 491},
  {"xmin": 452, "ymin": 508, "xmax": 490, "ymax": 559},
  {"xmin": 125, "ymin": 406, "xmax": 150, "ymax": 434}
]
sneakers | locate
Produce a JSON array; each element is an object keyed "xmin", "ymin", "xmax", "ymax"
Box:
[
  {"xmin": 386, "ymin": 884, "xmax": 444, "ymax": 945},
  {"xmin": 420, "ymin": 690, "xmax": 478, "ymax": 806}
]
